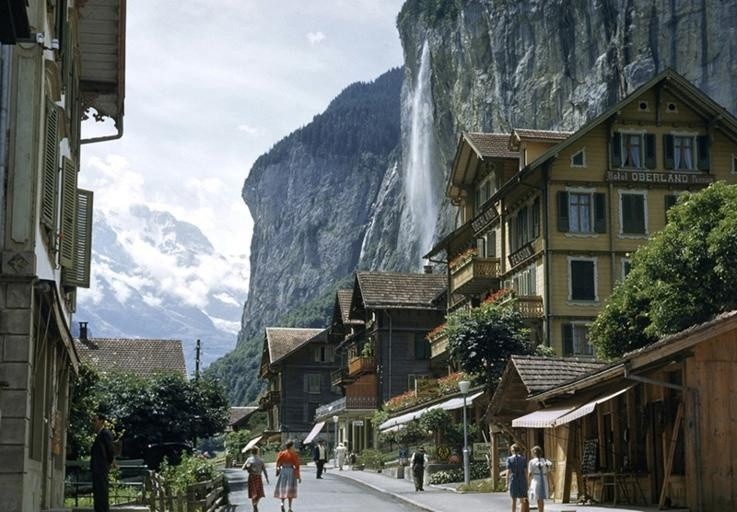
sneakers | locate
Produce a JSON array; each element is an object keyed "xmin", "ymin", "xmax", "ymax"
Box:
[
  {"xmin": 281, "ymin": 505, "xmax": 293, "ymax": 512},
  {"xmin": 416, "ymin": 487, "xmax": 424, "ymax": 491},
  {"xmin": 253, "ymin": 503, "xmax": 258, "ymax": 512}
]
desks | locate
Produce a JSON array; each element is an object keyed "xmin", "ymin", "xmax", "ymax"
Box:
[{"xmin": 573, "ymin": 471, "xmax": 630, "ymax": 507}]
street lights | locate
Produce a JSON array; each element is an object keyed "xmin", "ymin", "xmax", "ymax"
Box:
[{"xmin": 460, "ymin": 379, "xmax": 473, "ymax": 489}]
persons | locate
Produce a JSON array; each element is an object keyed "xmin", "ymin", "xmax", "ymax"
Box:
[
  {"xmin": 505, "ymin": 441, "xmax": 529, "ymax": 511},
  {"xmin": 526, "ymin": 444, "xmax": 554, "ymax": 511},
  {"xmin": 87, "ymin": 412, "xmax": 115, "ymax": 512},
  {"xmin": 313, "ymin": 439, "xmax": 324, "ymax": 479},
  {"xmin": 408, "ymin": 447, "xmax": 429, "ymax": 491},
  {"xmin": 333, "ymin": 441, "xmax": 348, "ymax": 472},
  {"xmin": 240, "ymin": 446, "xmax": 269, "ymax": 511},
  {"xmin": 272, "ymin": 439, "xmax": 302, "ymax": 511}
]
